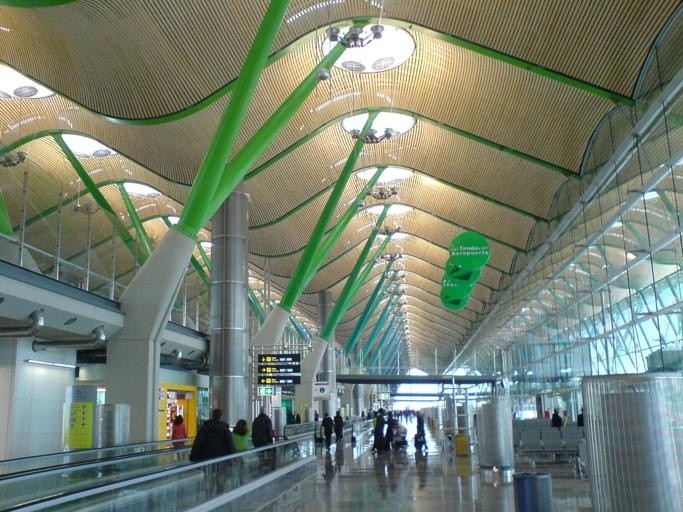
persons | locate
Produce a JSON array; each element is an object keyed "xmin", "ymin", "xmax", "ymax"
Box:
[
  {"xmin": 253, "ymin": 406, "xmax": 276, "ymax": 472},
  {"xmin": 562, "ymin": 411, "xmax": 569, "ymax": 428},
  {"xmin": 577, "ymin": 407, "xmax": 585, "ymax": 438},
  {"xmin": 230, "ymin": 418, "xmax": 253, "ymax": 487},
  {"xmin": 551, "ymin": 409, "xmax": 562, "ymax": 427},
  {"xmin": 293, "ymin": 408, "xmax": 428, "ymax": 452},
  {"xmin": 189, "ymin": 408, "xmax": 235, "ymax": 494},
  {"xmin": 170, "ymin": 415, "xmax": 189, "ymax": 460}
]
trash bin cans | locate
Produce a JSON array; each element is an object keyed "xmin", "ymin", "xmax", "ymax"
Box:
[
  {"xmin": 457, "ymin": 435, "xmax": 467, "ymax": 457},
  {"xmin": 513, "ymin": 472, "xmax": 552, "ymax": 512}
]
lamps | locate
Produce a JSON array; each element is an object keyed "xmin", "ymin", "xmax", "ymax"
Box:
[
  {"xmin": 61, "ymin": 134, "xmax": 120, "ymax": 159},
  {"xmin": 320, "ymin": 23, "xmax": 417, "ymax": 74},
  {"xmin": 0, "ymin": 64, "xmax": 57, "ymax": 99},
  {"xmin": 366, "ymin": 204, "xmax": 413, "ymax": 217},
  {"xmin": 341, "ymin": 112, "xmax": 418, "ymax": 135},
  {"xmin": 355, "ymin": 167, "xmax": 415, "ymax": 183},
  {"xmin": 376, "ymin": 232, "xmax": 412, "ymax": 360},
  {"xmin": 123, "ymin": 183, "xmax": 163, "ymax": 199}
]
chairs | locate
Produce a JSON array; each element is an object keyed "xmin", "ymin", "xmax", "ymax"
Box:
[{"xmin": 512, "ymin": 419, "xmax": 587, "ymax": 479}]
